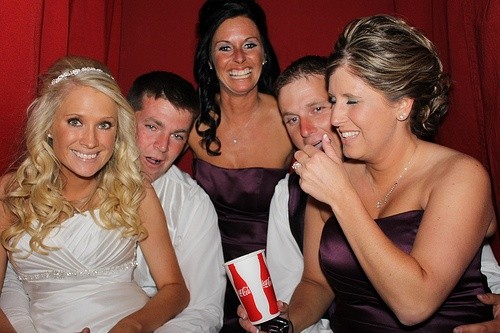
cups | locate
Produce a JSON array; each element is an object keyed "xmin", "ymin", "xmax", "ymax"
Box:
[{"xmin": 223, "ymin": 248, "xmax": 280, "ymax": 325}]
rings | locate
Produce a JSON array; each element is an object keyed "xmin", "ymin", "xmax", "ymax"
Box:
[{"xmin": 291, "ymin": 160, "xmax": 302, "ymax": 170}]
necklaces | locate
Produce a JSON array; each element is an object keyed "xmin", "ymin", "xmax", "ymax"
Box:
[
  {"xmin": 220, "ymin": 97, "xmax": 262, "ymax": 143},
  {"xmin": 365, "ymin": 140, "xmax": 424, "ymax": 208}
]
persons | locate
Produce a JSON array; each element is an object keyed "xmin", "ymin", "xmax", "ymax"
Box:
[
  {"xmin": 265, "ymin": 52, "xmax": 500, "ymax": 333},
  {"xmin": 1, "ymin": 54, "xmax": 190, "ymax": 333},
  {"xmin": 236, "ymin": 13, "xmax": 497, "ymax": 333},
  {"xmin": 178, "ymin": 0, "xmax": 301, "ymax": 332},
  {"xmin": 1, "ymin": 70, "xmax": 227, "ymax": 333}
]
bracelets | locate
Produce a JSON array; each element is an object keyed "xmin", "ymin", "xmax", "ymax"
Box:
[{"xmin": 290, "ymin": 320, "xmax": 294, "ymax": 333}]
